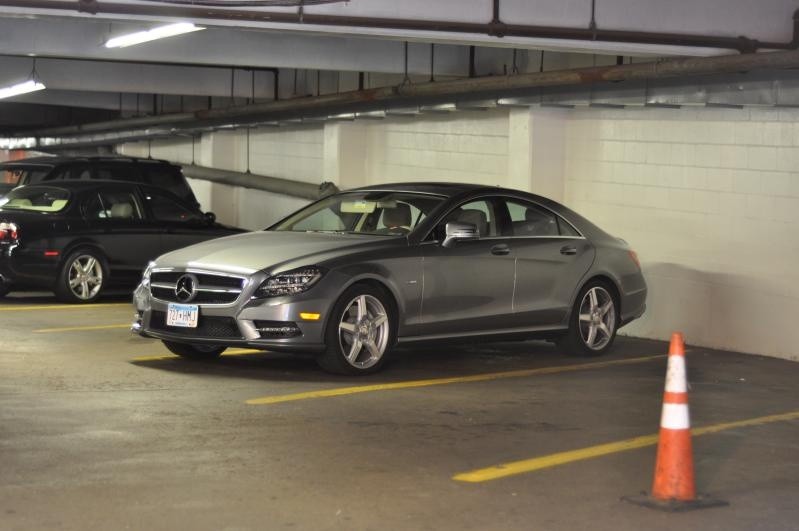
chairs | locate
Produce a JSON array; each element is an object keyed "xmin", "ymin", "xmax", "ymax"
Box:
[
  {"xmin": 445, "ymin": 208, "xmax": 556, "ymax": 241},
  {"xmin": 111, "ymin": 203, "xmax": 134, "ymax": 218},
  {"xmin": 374, "ymin": 203, "xmax": 412, "ymax": 235},
  {"xmin": 10, "ymin": 199, "xmax": 32, "ymax": 207}
]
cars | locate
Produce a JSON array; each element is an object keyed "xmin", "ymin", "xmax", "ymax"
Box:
[
  {"xmin": 0, "ymin": 179, "xmax": 254, "ymax": 305},
  {"xmin": 132, "ymin": 182, "xmax": 648, "ymax": 378}
]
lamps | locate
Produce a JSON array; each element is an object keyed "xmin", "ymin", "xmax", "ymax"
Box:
[{"xmin": 0, "ymin": 57, "xmax": 46, "ymax": 99}]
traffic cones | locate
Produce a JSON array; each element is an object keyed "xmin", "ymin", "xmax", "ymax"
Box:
[{"xmin": 619, "ymin": 331, "xmax": 731, "ymax": 514}]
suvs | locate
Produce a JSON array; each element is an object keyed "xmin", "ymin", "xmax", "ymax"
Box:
[{"xmin": 0, "ymin": 155, "xmax": 201, "ymax": 213}]
